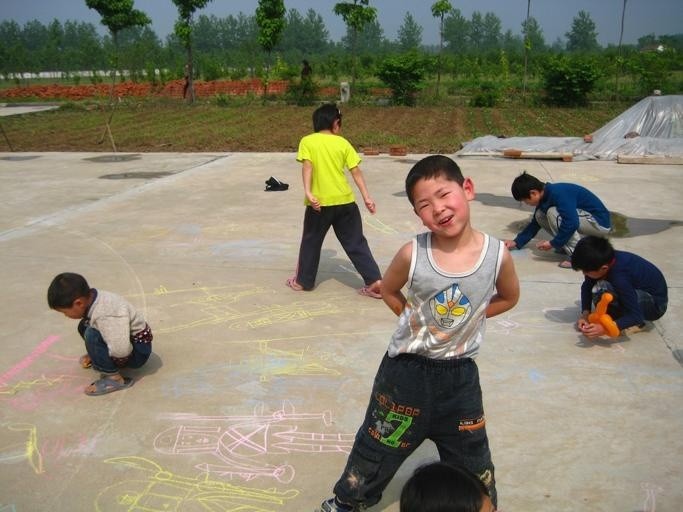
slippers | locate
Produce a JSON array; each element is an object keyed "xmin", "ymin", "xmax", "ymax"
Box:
[
  {"xmin": 78, "ymin": 353, "xmax": 96, "ymax": 370},
  {"xmin": 619, "ymin": 323, "xmax": 646, "ymax": 336},
  {"xmin": 357, "ymin": 286, "xmax": 383, "ymax": 299},
  {"xmin": 558, "ymin": 257, "xmax": 577, "ymax": 268},
  {"xmin": 85, "ymin": 376, "xmax": 133, "ymax": 396},
  {"xmin": 285, "ymin": 276, "xmax": 304, "ymax": 292}
]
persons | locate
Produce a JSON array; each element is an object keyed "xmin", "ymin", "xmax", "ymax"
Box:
[
  {"xmin": 286, "ymin": 103, "xmax": 382, "ymax": 301},
  {"xmin": 46, "ymin": 271, "xmax": 155, "ymax": 397},
  {"xmin": 399, "ymin": 459, "xmax": 497, "ymax": 512},
  {"xmin": 300, "ymin": 59, "xmax": 313, "ymax": 97},
  {"xmin": 335, "ymin": 154, "xmax": 522, "ymax": 512},
  {"xmin": 571, "ymin": 234, "xmax": 669, "ymax": 340},
  {"xmin": 182, "ymin": 63, "xmax": 191, "ymax": 98},
  {"xmin": 504, "ymin": 172, "xmax": 612, "ymax": 271}
]
